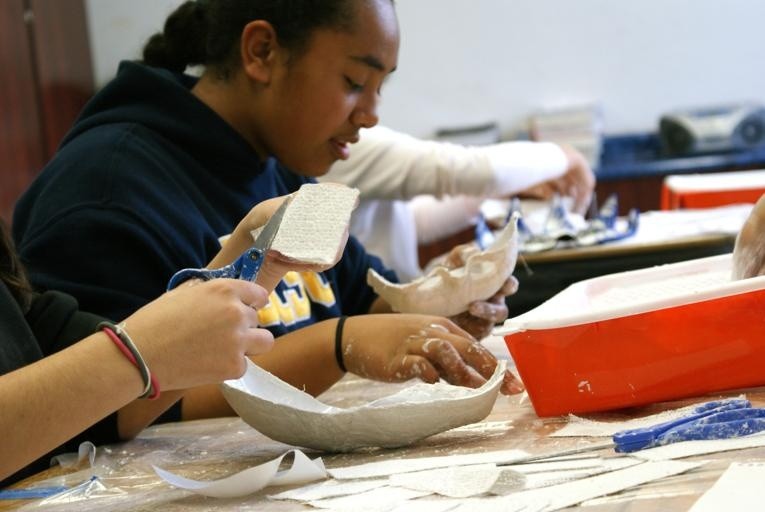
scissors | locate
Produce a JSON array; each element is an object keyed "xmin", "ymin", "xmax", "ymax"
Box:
[
  {"xmin": 167, "ymin": 195, "xmax": 299, "ymax": 295},
  {"xmin": 495, "ymin": 395, "xmax": 765, "ymax": 468}
]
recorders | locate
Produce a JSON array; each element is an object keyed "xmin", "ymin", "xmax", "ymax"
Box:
[{"xmin": 658, "ymin": 106, "xmax": 765, "ymax": 158}]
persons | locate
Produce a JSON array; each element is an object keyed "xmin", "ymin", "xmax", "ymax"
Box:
[
  {"xmin": 0, "ymin": 179, "xmax": 360, "ymax": 500},
  {"xmin": 731, "ymin": 190, "xmax": 765, "ymax": 279},
  {"xmin": 307, "ymin": 124, "xmax": 597, "ymax": 282},
  {"xmin": 12, "ymin": 0, "xmax": 527, "ymax": 398}
]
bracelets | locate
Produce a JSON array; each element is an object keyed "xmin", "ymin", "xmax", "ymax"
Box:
[
  {"xmin": 93, "ymin": 317, "xmax": 161, "ymax": 402},
  {"xmin": 336, "ymin": 315, "xmax": 347, "ymax": 373}
]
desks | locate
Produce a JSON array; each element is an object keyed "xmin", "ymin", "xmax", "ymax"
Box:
[{"xmin": 0, "ymin": 205, "xmax": 765, "ymax": 511}]
